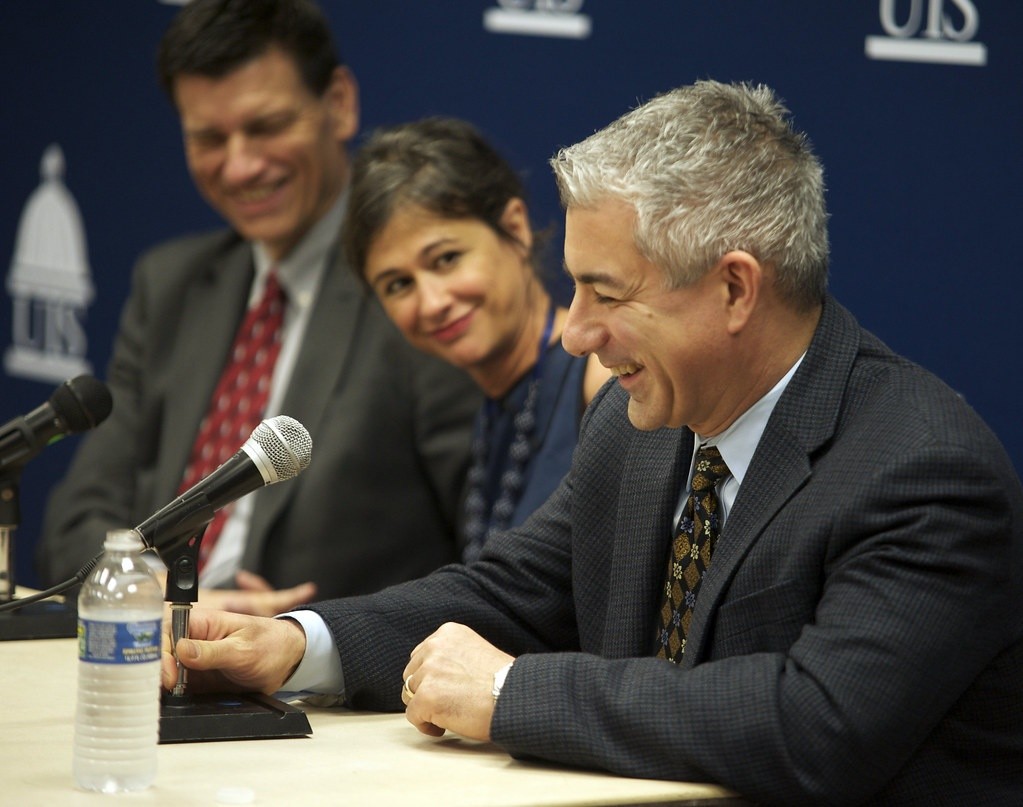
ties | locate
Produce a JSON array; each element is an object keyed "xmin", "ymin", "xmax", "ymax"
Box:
[
  {"xmin": 176, "ymin": 271, "xmax": 286, "ymax": 576},
  {"xmin": 651, "ymin": 444, "xmax": 732, "ymax": 664}
]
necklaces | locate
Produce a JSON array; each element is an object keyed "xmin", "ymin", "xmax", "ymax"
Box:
[{"xmin": 466, "ymin": 299, "xmax": 556, "ymax": 560}]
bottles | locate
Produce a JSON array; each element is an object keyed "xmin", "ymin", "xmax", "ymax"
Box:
[{"xmin": 74, "ymin": 529, "xmax": 164, "ymax": 792}]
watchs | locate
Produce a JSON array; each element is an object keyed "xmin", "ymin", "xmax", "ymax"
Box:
[{"xmin": 492, "ymin": 662, "xmax": 514, "ymax": 702}]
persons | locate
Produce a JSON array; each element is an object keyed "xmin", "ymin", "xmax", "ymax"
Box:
[
  {"xmin": 344, "ymin": 117, "xmax": 611, "ymax": 562},
  {"xmin": 161, "ymin": 79, "xmax": 1023, "ymax": 807},
  {"xmin": 38, "ymin": 0, "xmax": 490, "ymax": 615}
]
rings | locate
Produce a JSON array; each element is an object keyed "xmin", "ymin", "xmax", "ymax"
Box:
[{"xmin": 404, "ymin": 675, "xmax": 415, "ymax": 698}]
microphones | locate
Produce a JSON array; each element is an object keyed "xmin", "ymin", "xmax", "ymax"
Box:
[
  {"xmin": 80, "ymin": 416, "xmax": 314, "ymax": 582},
  {"xmin": 0, "ymin": 375, "xmax": 114, "ymax": 470}
]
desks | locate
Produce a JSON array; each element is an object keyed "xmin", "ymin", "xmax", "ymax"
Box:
[{"xmin": 0, "ymin": 640, "xmax": 726, "ymax": 807}]
